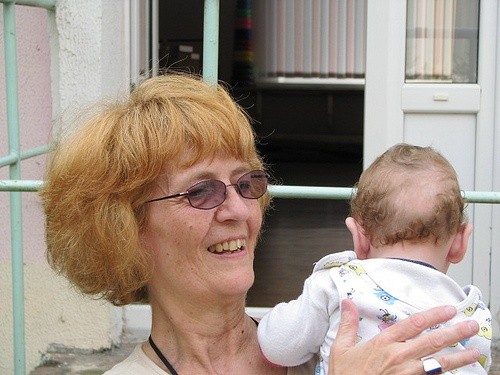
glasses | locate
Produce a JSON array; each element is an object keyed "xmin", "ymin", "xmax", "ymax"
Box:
[{"xmin": 144, "ymin": 169, "xmax": 270, "ymax": 210}]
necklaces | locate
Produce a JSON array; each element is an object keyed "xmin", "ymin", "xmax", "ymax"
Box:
[{"xmin": 148, "ymin": 316, "xmax": 259, "ymax": 375}]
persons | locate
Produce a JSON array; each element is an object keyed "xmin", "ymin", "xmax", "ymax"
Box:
[
  {"xmin": 38, "ymin": 57, "xmax": 480, "ymax": 375},
  {"xmin": 257, "ymin": 143, "xmax": 492, "ymax": 375}
]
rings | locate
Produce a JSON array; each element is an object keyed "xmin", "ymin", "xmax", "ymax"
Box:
[{"xmin": 420, "ymin": 356, "xmax": 443, "ymax": 375}]
